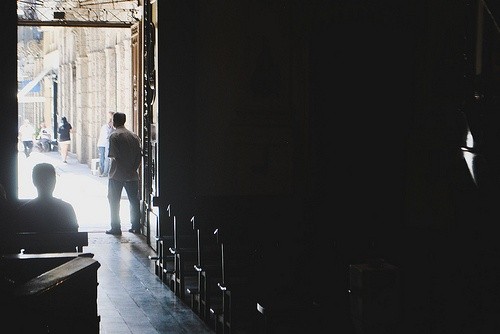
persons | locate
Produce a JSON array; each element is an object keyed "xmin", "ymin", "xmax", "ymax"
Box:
[
  {"xmin": 106, "ymin": 113, "xmax": 142, "ymax": 234},
  {"xmin": 21, "ymin": 116, "xmax": 115, "ymax": 177},
  {"xmin": 15, "ymin": 163, "xmax": 79, "ymax": 253}
]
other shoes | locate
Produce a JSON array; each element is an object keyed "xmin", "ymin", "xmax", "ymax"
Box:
[
  {"xmin": 106, "ymin": 229, "xmax": 121, "ymax": 235},
  {"xmin": 129, "ymin": 229, "xmax": 140, "ymax": 233}
]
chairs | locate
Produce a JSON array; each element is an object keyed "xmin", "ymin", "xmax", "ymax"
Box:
[{"xmin": 18, "ymin": 231, "xmax": 88, "ymax": 254}]
